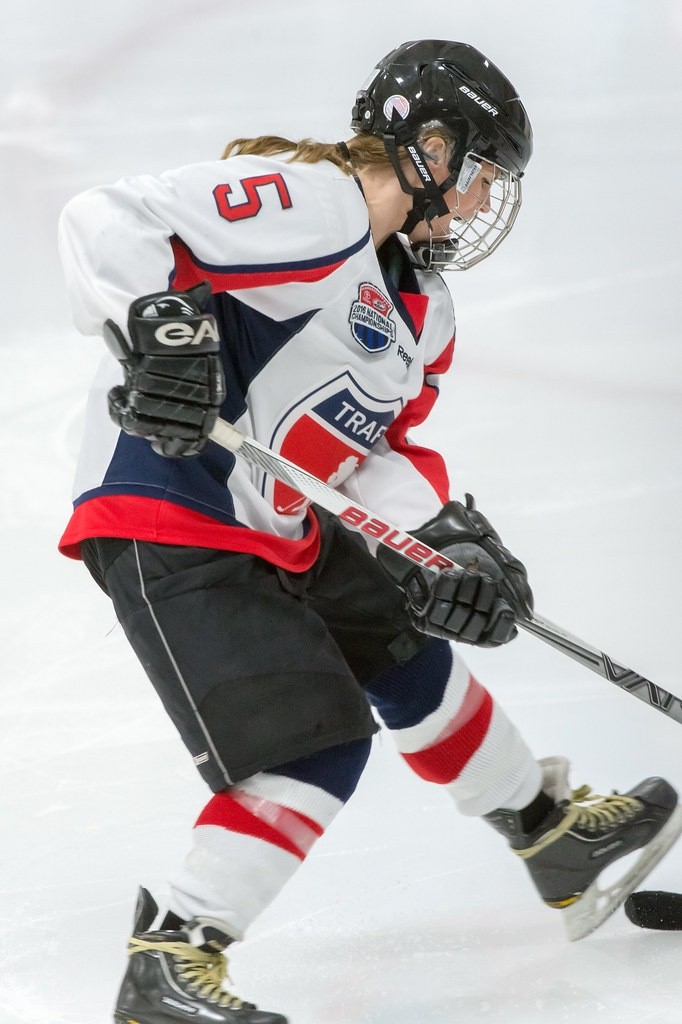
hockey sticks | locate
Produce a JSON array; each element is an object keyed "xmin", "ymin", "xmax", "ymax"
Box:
[{"xmin": 206, "ymin": 418, "xmax": 682, "ymax": 932}]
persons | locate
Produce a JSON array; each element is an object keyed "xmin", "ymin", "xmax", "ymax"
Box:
[{"xmin": 54, "ymin": 37, "xmax": 680, "ymax": 1023}]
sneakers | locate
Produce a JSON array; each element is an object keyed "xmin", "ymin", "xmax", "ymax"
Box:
[
  {"xmin": 483, "ymin": 757, "xmax": 682, "ymax": 942},
  {"xmin": 113, "ymin": 884, "xmax": 289, "ymax": 1023}
]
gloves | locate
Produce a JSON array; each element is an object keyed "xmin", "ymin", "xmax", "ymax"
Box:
[
  {"xmin": 376, "ymin": 493, "xmax": 534, "ymax": 647},
  {"xmin": 101, "ymin": 279, "xmax": 227, "ymax": 459}
]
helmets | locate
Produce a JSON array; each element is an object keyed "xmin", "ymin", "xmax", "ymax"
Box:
[{"xmin": 349, "ymin": 40, "xmax": 534, "ymax": 272}]
